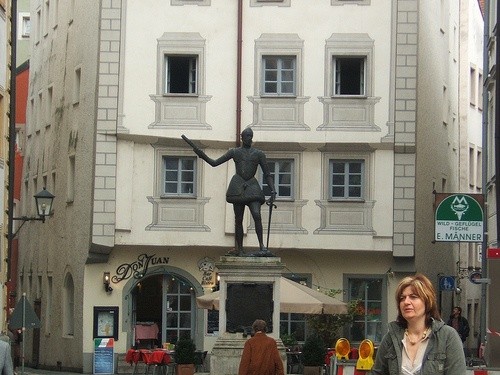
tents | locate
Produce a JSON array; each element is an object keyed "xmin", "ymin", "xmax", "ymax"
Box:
[{"xmin": 196, "ymin": 275, "xmax": 349, "ymax": 367}]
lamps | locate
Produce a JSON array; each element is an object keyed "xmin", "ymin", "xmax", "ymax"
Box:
[
  {"xmin": 103, "ymin": 271, "xmax": 112, "ymax": 292},
  {"xmin": 212, "ymin": 272, "xmax": 220, "ymax": 292}
]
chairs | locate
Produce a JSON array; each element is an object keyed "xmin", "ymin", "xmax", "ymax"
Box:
[{"xmin": 125, "ymin": 349, "xmax": 170, "ymax": 375}]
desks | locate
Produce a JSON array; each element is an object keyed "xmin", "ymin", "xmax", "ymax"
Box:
[{"xmin": 135, "ymin": 324, "xmax": 159, "ymax": 349}]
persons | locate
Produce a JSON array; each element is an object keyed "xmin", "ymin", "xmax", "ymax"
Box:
[
  {"xmin": 447, "ymin": 305, "xmax": 470, "ymax": 344},
  {"xmin": 174, "ymin": 330, "xmax": 195, "ymax": 375},
  {"xmin": 238, "ymin": 319, "xmax": 285, "ymax": 375},
  {"xmin": 0, "ymin": 341, "xmax": 13, "ymax": 375},
  {"xmin": 182, "ymin": 126, "xmax": 276, "ymax": 255},
  {"xmin": 370, "ymin": 274, "xmax": 468, "ymax": 375}
]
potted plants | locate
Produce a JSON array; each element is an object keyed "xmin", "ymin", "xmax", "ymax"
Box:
[
  {"xmin": 175, "ymin": 333, "xmax": 196, "ymax": 375},
  {"xmin": 303, "ymin": 305, "xmax": 351, "ymax": 375}
]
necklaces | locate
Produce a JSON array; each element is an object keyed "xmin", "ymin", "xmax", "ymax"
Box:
[{"xmin": 404, "ymin": 326, "xmax": 428, "ymax": 344}]
